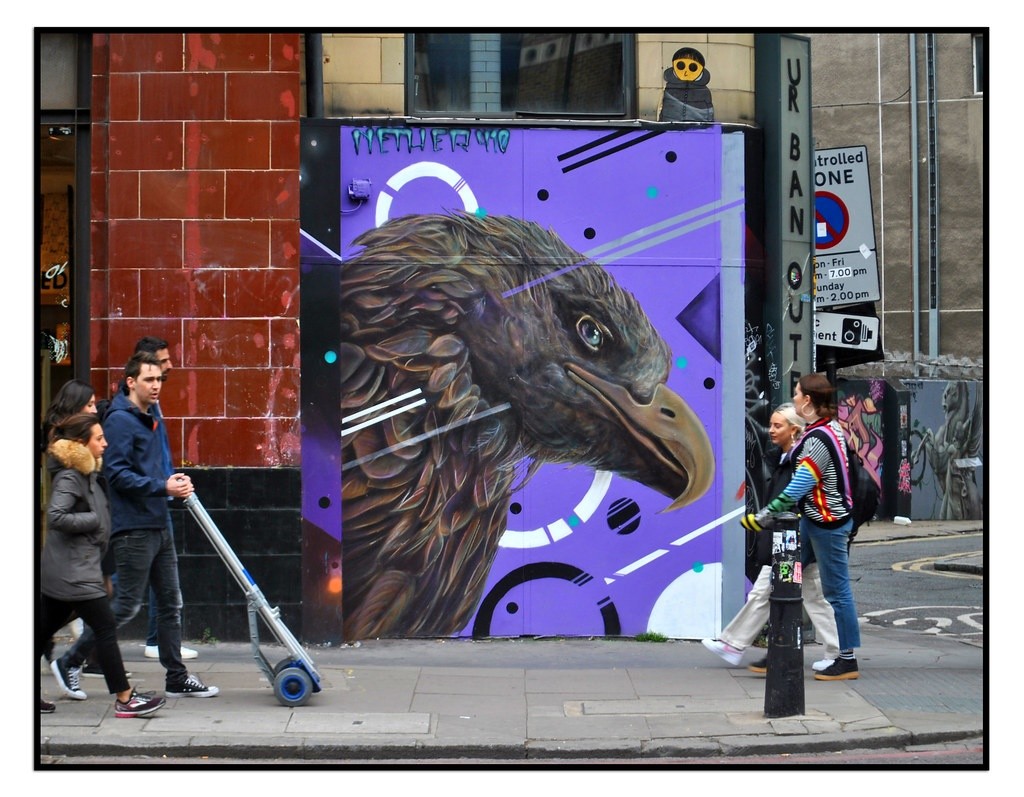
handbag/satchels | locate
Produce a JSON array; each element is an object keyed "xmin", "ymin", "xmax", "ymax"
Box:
[{"xmin": 845, "ymin": 449, "xmax": 881, "ymax": 534}]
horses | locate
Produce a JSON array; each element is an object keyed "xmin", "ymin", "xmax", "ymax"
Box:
[{"xmin": 912, "ymin": 381, "xmax": 982, "ymax": 521}]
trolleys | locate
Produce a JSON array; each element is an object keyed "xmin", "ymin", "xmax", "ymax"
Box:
[{"xmin": 168, "ymin": 476, "xmax": 327, "ymax": 708}]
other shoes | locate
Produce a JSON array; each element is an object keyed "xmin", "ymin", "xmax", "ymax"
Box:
[{"xmin": 82, "ymin": 660, "xmax": 131, "ymax": 677}]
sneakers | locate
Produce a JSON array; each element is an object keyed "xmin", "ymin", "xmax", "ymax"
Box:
[
  {"xmin": 814, "ymin": 657, "xmax": 859, "ymax": 681},
  {"xmin": 69, "ymin": 616, "xmax": 83, "ymax": 640},
  {"xmin": 749, "ymin": 656, "xmax": 768, "ymax": 672},
  {"xmin": 165, "ymin": 673, "xmax": 219, "ymax": 697},
  {"xmin": 39, "ymin": 699, "xmax": 56, "ymax": 713},
  {"xmin": 702, "ymin": 638, "xmax": 744, "ymax": 665},
  {"xmin": 50, "ymin": 658, "xmax": 87, "ymax": 699},
  {"xmin": 812, "ymin": 659, "xmax": 835, "ymax": 670},
  {"xmin": 115, "ymin": 688, "xmax": 167, "ymax": 717},
  {"xmin": 144, "ymin": 643, "xmax": 198, "ymax": 659}
]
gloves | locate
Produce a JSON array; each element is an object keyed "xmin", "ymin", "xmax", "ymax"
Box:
[{"xmin": 739, "ymin": 512, "xmax": 763, "ymax": 531}]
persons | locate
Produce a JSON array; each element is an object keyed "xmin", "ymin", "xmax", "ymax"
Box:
[
  {"xmin": 742, "ymin": 372, "xmax": 861, "ymax": 680},
  {"xmin": 702, "ymin": 402, "xmax": 840, "ymax": 670},
  {"xmin": 39, "ymin": 337, "xmax": 220, "ymax": 718}
]
